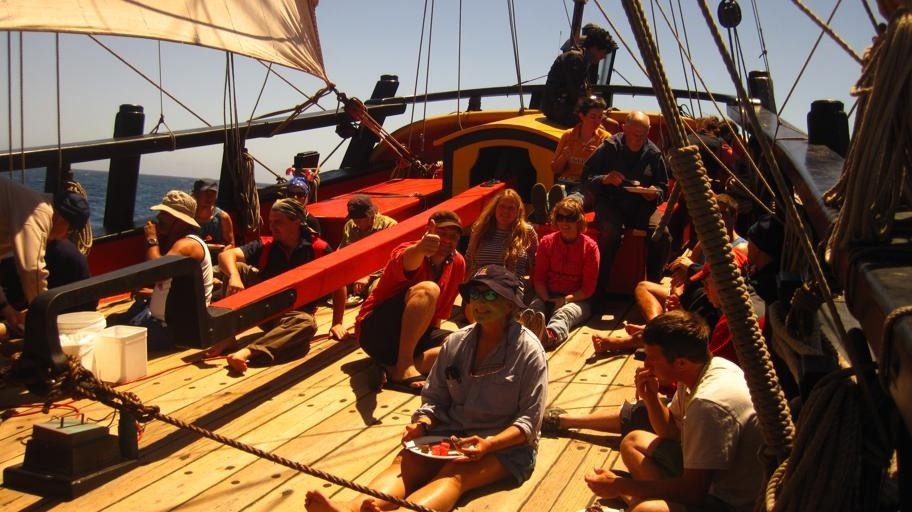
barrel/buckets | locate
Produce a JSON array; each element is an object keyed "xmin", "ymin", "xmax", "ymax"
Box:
[{"xmin": 57, "ymin": 308, "xmax": 107, "ymax": 380}]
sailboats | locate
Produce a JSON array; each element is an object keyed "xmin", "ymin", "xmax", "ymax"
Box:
[{"xmin": 0, "ymin": 0, "xmax": 911, "ymax": 510}]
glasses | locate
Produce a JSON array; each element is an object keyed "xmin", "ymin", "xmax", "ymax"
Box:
[
  {"xmin": 287, "ymin": 192, "xmax": 304, "ymax": 197},
  {"xmin": 469, "ymin": 288, "xmax": 495, "ymax": 302}
]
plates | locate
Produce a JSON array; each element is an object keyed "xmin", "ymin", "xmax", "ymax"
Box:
[
  {"xmin": 406, "ymin": 436, "xmax": 475, "ymax": 459},
  {"xmin": 624, "ymin": 186, "xmax": 662, "ymax": 193}
]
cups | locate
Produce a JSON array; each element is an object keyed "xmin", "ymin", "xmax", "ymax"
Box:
[{"xmin": 133, "ymin": 214, "xmax": 158, "ymax": 226}]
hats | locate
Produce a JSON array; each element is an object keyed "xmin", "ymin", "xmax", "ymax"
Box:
[
  {"xmin": 748, "ymin": 214, "xmax": 780, "ymax": 254},
  {"xmin": 690, "ymin": 249, "xmax": 749, "ymax": 282},
  {"xmin": 151, "ymin": 189, "xmax": 201, "ymax": 227},
  {"xmin": 428, "ymin": 211, "xmax": 463, "ymax": 231},
  {"xmin": 458, "ymin": 265, "xmax": 530, "ymax": 308},
  {"xmin": 583, "ymin": 28, "xmax": 619, "ymax": 50},
  {"xmin": 345, "ymin": 196, "xmax": 371, "ymax": 218},
  {"xmin": 194, "ymin": 180, "xmax": 218, "ymax": 192},
  {"xmin": 53, "ymin": 191, "xmax": 90, "ymax": 224}
]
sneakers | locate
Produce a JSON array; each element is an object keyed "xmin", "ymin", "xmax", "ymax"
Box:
[
  {"xmin": 530, "ymin": 183, "xmax": 547, "ymax": 225},
  {"xmin": 541, "ymin": 408, "xmax": 565, "ymax": 432},
  {"xmin": 549, "ymin": 185, "xmax": 564, "ymax": 212},
  {"xmin": 521, "ymin": 309, "xmax": 549, "ymax": 350}
]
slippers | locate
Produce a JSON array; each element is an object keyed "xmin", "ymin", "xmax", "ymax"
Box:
[{"xmin": 384, "ymin": 375, "xmax": 426, "ymax": 395}]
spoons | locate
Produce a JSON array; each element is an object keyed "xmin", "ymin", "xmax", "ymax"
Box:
[{"xmin": 623, "ymin": 178, "xmax": 640, "ymax": 186}]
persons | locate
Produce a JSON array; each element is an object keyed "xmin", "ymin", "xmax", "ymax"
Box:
[
  {"xmin": 536, "ymin": 21, "xmax": 622, "ymax": 131},
  {"xmin": 0, "ymin": 94, "xmax": 782, "ymax": 512}
]
